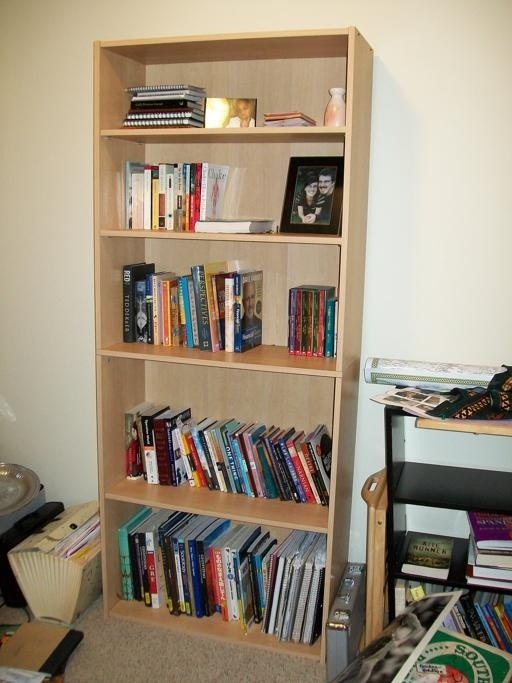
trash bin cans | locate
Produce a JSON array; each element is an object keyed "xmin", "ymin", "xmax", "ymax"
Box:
[{"xmin": 1, "ymin": 462, "xmax": 46, "ymax": 608}]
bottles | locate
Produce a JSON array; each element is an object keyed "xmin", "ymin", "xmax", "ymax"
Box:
[{"xmin": 324, "ymin": 88, "xmax": 346, "ymax": 127}]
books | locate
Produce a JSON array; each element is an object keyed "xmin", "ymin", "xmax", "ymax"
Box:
[
  {"xmin": 0, "ymin": 622, "xmax": 85, "ymax": 678},
  {"xmin": 415, "ymin": 417, "xmax": 512, "ymax": 437},
  {"xmin": 393, "ymin": 511, "xmax": 512, "ymax": 683}
]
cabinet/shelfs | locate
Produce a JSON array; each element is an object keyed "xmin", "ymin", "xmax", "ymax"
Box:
[
  {"xmin": 384, "ymin": 397, "xmax": 512, "ymax": 626},
  {"xmin": 89, "ymin": 25, "xmax": 375, "ymax": 667}
]
